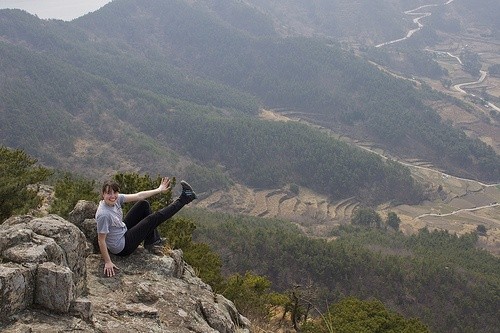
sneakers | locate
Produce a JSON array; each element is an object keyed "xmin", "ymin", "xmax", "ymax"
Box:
[
  {"xmin": 144, "ymin": 238, "xmax": 165, "ymax": 249},
  {"xmin": 181, "ymin": 180, "xmax": 198, "ymax": 200}
]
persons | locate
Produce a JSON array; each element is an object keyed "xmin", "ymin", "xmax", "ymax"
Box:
[{"xmin": 95, "ymin": 174, "xmax": 200, "ymax": 278}]
